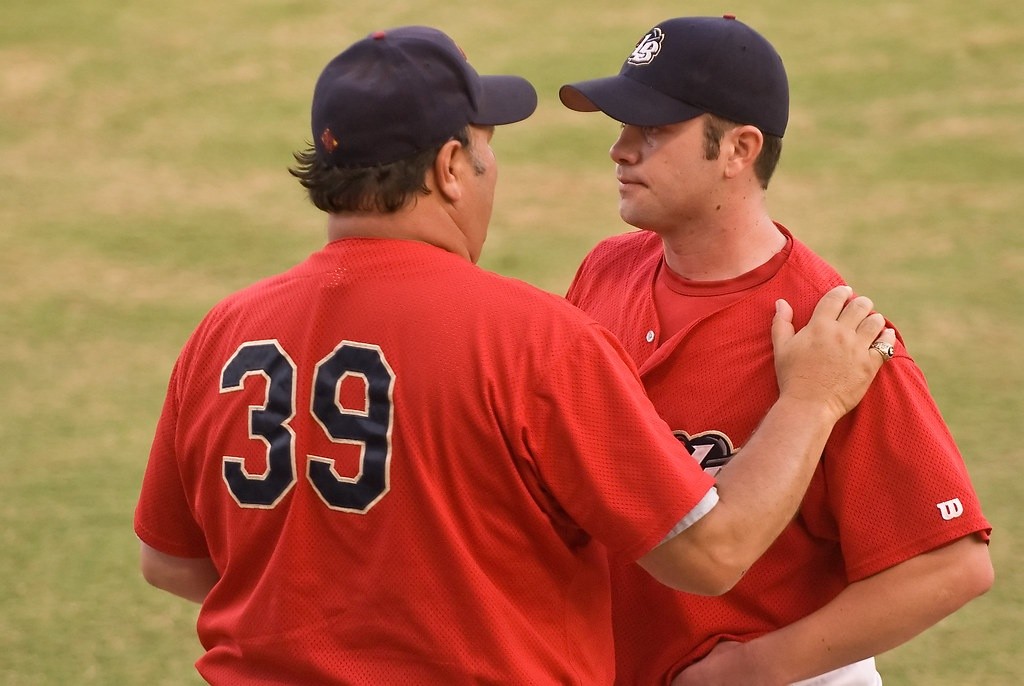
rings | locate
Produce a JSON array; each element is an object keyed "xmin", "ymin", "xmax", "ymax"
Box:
[{"xmin": 871, "ymin": 341, "xmax": 893, "ymax": 361}]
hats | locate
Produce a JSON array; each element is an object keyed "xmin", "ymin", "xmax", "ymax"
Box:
[
  {"xmin": 560, "ymin": 14, "xmax": 788, "ymax": 138},
  {"xmin": 311, "ymin": 25, "xmax": 537, "ymax": 168}
]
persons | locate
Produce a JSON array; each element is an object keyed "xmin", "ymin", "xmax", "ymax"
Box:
[
  {"xmin": 558, "ymin": 14, "xmax": 993, "ymax": 684},
  {"xmin": 135, "ymin": 27, "xmax": 897, "ymax": 686}
]
